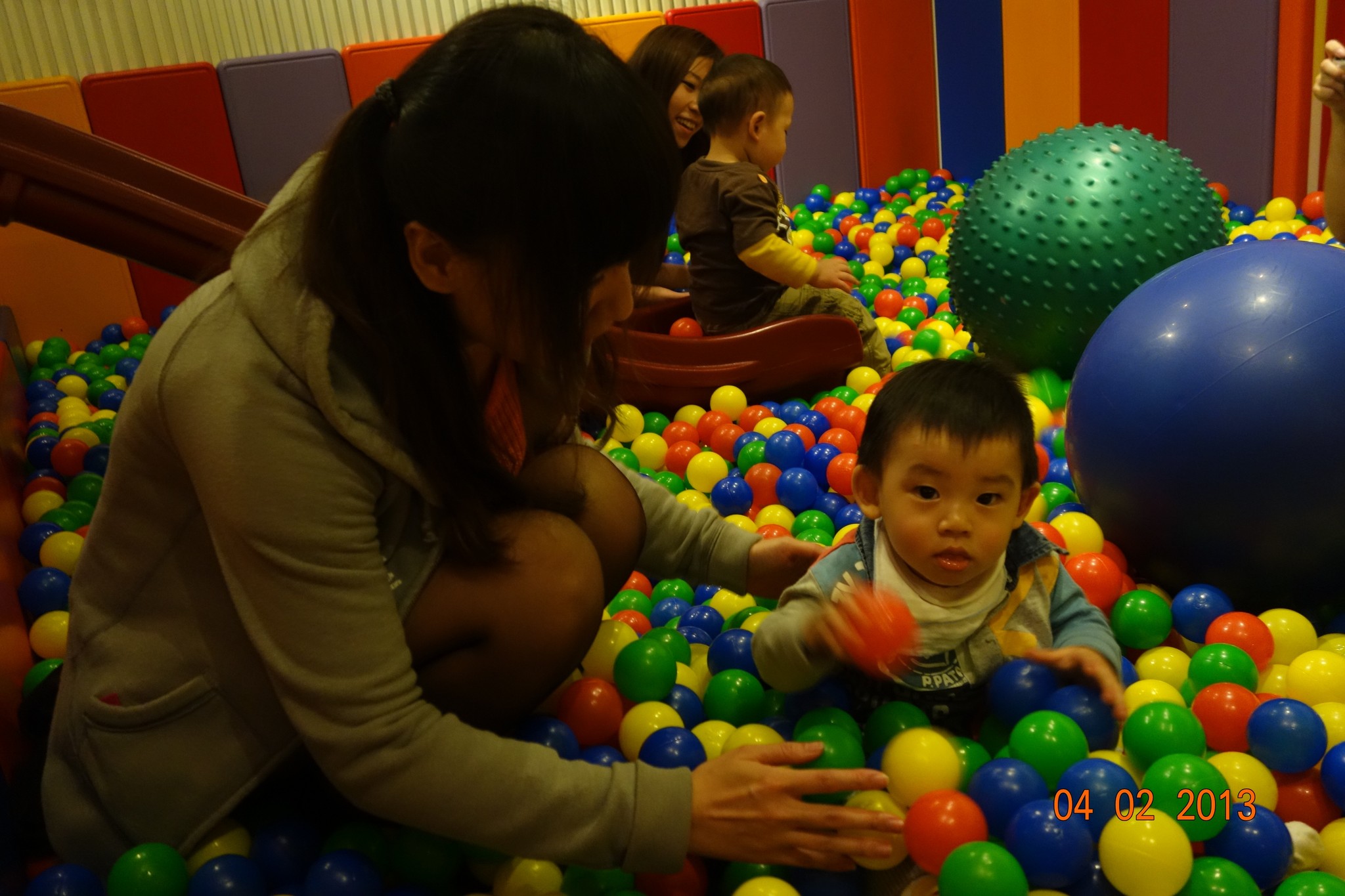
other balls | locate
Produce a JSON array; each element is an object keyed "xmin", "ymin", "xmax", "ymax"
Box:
[
  {"xmin": 13, "ymin": 167, "xmax": 1345, "ymax": 896},
  {"xmin": 946, "ymin": 121, "xmax": 1233, "ymax": 382},
  {"xmin": 1063, "ymin": 239, "xmax": 1345, "ymax": 637}
]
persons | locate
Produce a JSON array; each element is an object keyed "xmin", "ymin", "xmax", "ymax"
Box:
[
  {"xmin": 674, "ymin": 53, "xmax": 891, "ymax": 380},
  {"xmin": 750, "ymin": 360, "xmax": 1128, "ymax": 737},
  {"xmin": 629, "ymin": 24, "xmax": 720, "ymax": 308},
  {"xmin": 38, "ymin": 1, "xmax": 902, "ymax": 871},
  {"xmin": 1310, "ymin": 40, "xmax": 1345, "ymax": 244}
]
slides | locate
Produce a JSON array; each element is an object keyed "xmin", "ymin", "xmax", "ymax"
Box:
[{"xmin": 1, "ymin": 103, "xmax": 864, "ymax": 403}]
